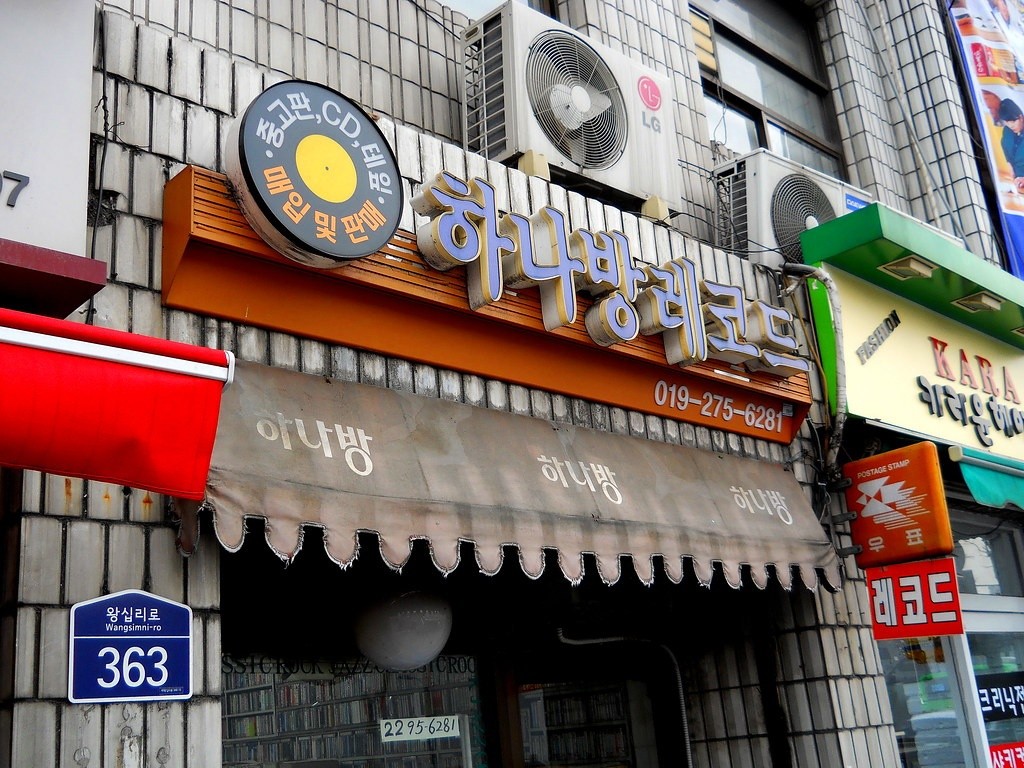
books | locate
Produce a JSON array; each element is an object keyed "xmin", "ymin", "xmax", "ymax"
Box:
[
  {"xmin": 224, "ymin": 655, "xmax": 477, "ymax": 768},
  {"xmin": 520, "ymin": 691, "xmax": 628, "ymax": 763}
]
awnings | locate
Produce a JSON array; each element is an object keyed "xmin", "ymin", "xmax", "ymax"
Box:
[
  {"xmin": 171, "ymin": 358, "xmax": 846, "ymax": 592},
  {"xmin": 0, "ymin": 307, "xmax": 235, "ymax": 501},
  {"xmin": 948, "ymin": 446, "xmax": 1023, "ymax": 509}
]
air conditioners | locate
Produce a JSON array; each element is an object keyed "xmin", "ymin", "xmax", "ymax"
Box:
[
  {"xmin": 459, "ymin": 0, "xmax": 684, "ymax": 217},
  {"xmin": 712, "ymin": 147, "xmax": 872, "ymax": 275}
]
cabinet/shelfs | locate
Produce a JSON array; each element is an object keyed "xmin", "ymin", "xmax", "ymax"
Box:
[{"xmin": 220, "ymin": 661, "xmax": 634, "ymax": 768}]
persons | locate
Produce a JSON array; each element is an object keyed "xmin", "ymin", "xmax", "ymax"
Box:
[
  {"xmin": 981, "ymin": 89, "xmax": 1014, "ymax": 176},
  {"xmin": 994, "ymin": 0, "xmax": 1024, "ymax": 34},
  {"xmin": 998, "ymin": 98, "xmax": 1024, "ymax": 193}
]
